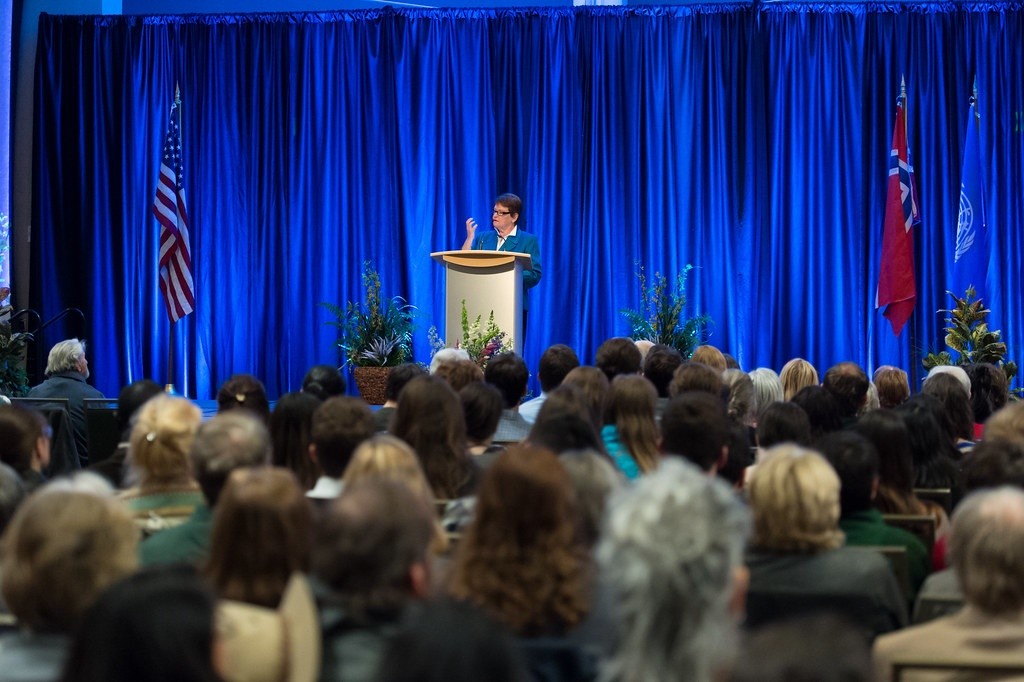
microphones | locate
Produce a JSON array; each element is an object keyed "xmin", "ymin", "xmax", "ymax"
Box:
[{"xmin": 480, "ymin": 220, "xmax": 494, "ymax": 250}]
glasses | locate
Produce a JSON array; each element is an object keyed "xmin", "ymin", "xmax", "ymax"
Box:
[{"xmin": 492, "ymin": 210, "xmax": 512, "ymax": 215}]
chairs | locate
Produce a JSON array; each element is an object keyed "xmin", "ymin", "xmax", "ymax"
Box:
[
  {"xmin": 434, "ymin": 441, "xmax": 1024, "ymax": 682},
  {"xmin": 8, "ymin": 397, "xmax": 81, "ymax": 479},
  {"xmin": 82, "ymin": 398, "xmax": 121, "ymax": 465}
]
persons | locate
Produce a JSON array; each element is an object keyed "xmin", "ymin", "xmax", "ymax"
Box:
[
  {"xmin": 0, "ymin": 338, "xmax": 1024, "ymax": 682},
  {"xmin": 462, "ymin": 194, "xmax": 542, "ymax": 358}
]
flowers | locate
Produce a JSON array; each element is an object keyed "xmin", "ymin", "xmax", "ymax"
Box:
[
  {"xmin": 317, "ymin": 258, "xmax": 419, "ymax": 374},
  {"xmin": 417, "ymin": 298, "xmax": 513, "ymax": 377}
]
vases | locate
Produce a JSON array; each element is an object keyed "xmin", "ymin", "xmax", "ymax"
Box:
[{"xmin": 353, "ymin": 366, "xmax": 401, "ymax": 404}]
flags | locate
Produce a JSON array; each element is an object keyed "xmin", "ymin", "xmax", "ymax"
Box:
[
  {"xmin": 947, "ymin": 102, "xmax": 991, "ymax": 363},
  {"xmin": 875, "ymin": 97, "xmax": 921, "ymax": 337},
  {"xmin": 152, "ymin": 102, "xmax": 195, "ymax": 325}
]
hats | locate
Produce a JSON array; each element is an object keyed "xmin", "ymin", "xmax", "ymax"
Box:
[{"xmin": 925, "ymin": 365, "xmax": 972, "ymax": 401}]
